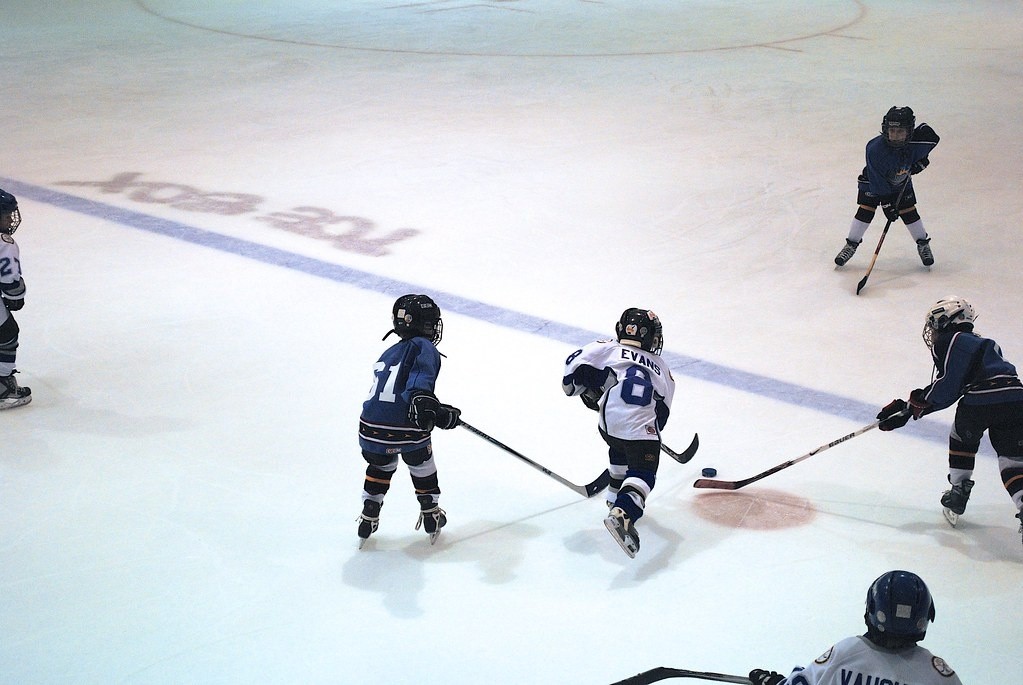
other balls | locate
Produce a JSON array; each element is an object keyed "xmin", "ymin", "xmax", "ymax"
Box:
[{"xmin": 702, "ymin": 468, "xmax": 717, "ymax": 478}]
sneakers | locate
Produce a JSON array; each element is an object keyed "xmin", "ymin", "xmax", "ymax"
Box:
[
  {"xmin": 355, "ymin": 498, "xmax": 383, "ymax": 550},
  {"xmin": 917, "ymin": 233, "xmax": 934, "ymax": 272},
  {"xmin": 1015, "ymin": 495, "xmax": 1023, "ymax": 545},
  {"xmin": 415, "ymin": 494, "xmax": 447, "ymax": 545},
  {"xmin": 604, "ymin": 500, "xmax": 640, "ymax": 559},
  {"xmin": 0, "ymin": 370, "xmax": 32, "ymax": 410},
  {"xmin": 833, "ymin": 237, "xmax": 863, "ymax": 270},
  {"xmin": 941, "ymin": 479, "xmax": 975, "ymax": 528}
]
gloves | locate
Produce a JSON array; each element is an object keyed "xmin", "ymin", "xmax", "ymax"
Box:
[
  {"xmin": 910, "ymin": 389, "xmax": 931, "ymax": 420},
  {"xmin": 579, "ymin": 386, "xmax": 604, "ymax": 413},
  {"xmin": 435, "ymin": 404, "xmax": 461, "ymax": 431},
  {"xmin": 909, "ymin": 158, "xmax": 930, "ymax": 175},
  {"xmin": 876, "ymin": 399, "xmax": 912, "ymax": 431},
  {"xmin": 881, "ymin": 197, "xmax": 899, "ymax": 222},
  {"xmin": 4, "ymin": 297, "xmax": 24, "ymax": 310},
  {"xmin": 748, "ymin": 668, "xmax": 786, "ymax": 685},
  {"xmin": 407, "ymin": 390, "xmax": 440, "ymax": 432}
]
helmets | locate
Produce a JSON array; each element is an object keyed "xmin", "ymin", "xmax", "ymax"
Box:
[
  {"xmin": 0, "ymin": 189, "xmax": 21, "ymax": 235},
  {"xmin": 882, "ymin": 105, "xmax": 916, "ymax": 151},
  {"xmin": 922, "ymin": 295, "xmax": 975, "ymax": 350},
  {"xmin": 393, "ymin": 293, "xmax": 443, "ymax": 347},
  {"xmin": 864, "ymin": 570, "xmax": 935, "ymax": 641},
  {"xmin": 615, "ymin": 308, "xmax": 663, "ymax": 356}
]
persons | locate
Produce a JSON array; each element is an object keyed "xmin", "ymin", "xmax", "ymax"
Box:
[
  {"xmin": 563, "ymin": 306, "xmax": 676, "ymax": 559},
  {"xmin": 876, "ymin": 294, "xmax": 1023, "ymax": 537},
  {"xmin": 355, "ymin": 293, "xmax": 461, "ymax": 549},
  {"xmin": 749, "ymin": 570, "xmax": 963, "ymax": 685},
  {"xmin": 834, "ymin": 105, "xmax": 941, "ymax": 271},
  {"xmin": 0, "ymin": 188, "xmax": 32, "ymax": 411}
]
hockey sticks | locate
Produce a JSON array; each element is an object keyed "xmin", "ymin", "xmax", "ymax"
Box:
[
  {"xmin": 855, "ymin": 171, "xmax": 914, "ymax": 296},
  {"xmin": 583, "ymin": 389, "xmax": 699, "ymax": 465},
  {"xmin": 692, "ymin": 408, "xmax": 908, "ymax": 490},
  {"xmin": 609, "ymin": 666, "xmax": 752, "ymax": 685},
  {"xmin": 459, "ymin": 419, "xmax": 611, "ymax": 499}
]
